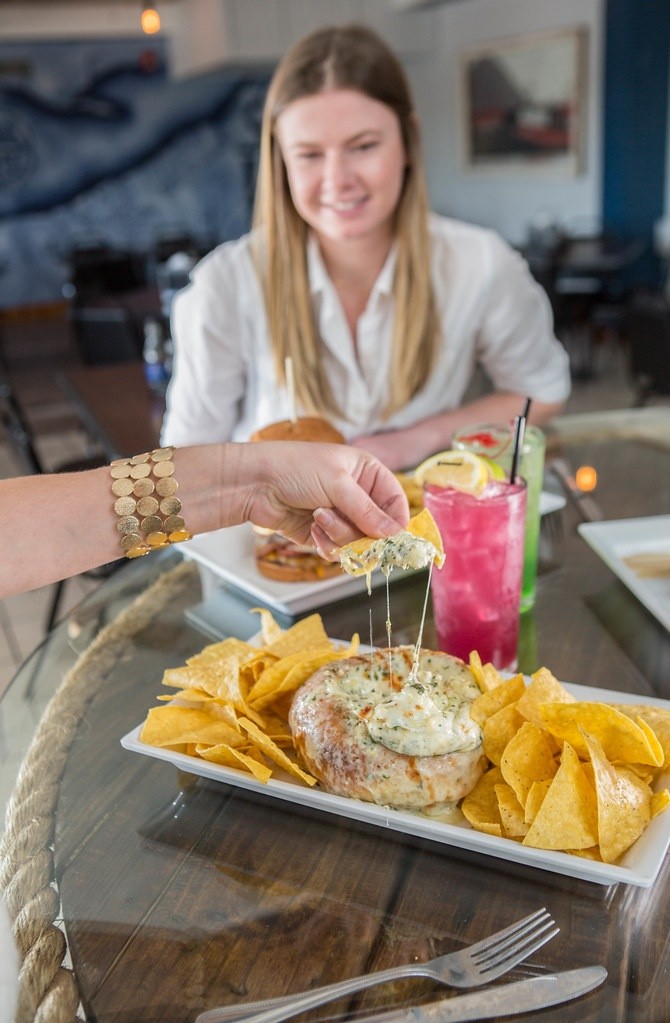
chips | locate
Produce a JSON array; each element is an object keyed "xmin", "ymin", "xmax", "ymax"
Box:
[
  {"xmin": 139, "ymin": 608, "xmax": 364, "ymax": 787},
  {"xmin": 330, "ymin": 505, "xmax": 446, "ymax": 571},
  {"xmin": 463, "ymin": 649, "xmax": 670, "ymax": 862}
]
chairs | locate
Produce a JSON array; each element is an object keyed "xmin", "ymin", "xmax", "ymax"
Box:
[{"xmin": 3, "ymin": 222, "xmax": 670, "ymax": 701}]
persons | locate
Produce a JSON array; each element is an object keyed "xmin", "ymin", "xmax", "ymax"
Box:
[
  {"xmin": 1, "ymin": 439, "xmax": 411, "ymax": 599},
  {"xmin": 161, "ymin": 21, "xmax": 574, "ymax": 476}
]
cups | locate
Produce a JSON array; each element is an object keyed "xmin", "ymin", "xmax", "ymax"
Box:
[
  {"xmin": 423, "ymin": 470, "xmax": 528, "ymax": 671},
  {"xmin": 450, "ymin": 418, "xmax": 545, "ymax": 612}
]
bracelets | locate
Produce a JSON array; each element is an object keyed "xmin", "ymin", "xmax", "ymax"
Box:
[{"xmin": 112, "ymin": 444, "xmax": 192, "ymax": 559}]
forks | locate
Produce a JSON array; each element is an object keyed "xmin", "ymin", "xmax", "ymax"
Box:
[{"xmin": 194, "ymin": 904, "xmax": 560, "ymax": 1023}]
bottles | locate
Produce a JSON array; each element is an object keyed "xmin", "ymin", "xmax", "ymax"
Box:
[{"xmin": 141, "ymin": 316, "xmax": 173, "ymax": 396}]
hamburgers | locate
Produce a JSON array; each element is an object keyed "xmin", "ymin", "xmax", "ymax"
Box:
[{"xmin": 248, "ymin": 417, "xmax": 348, "ymax": 578}]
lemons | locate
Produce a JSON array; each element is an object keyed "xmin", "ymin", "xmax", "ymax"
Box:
[
  {"xmin": 413, "ymin": 450, "xmax": 489, "ymax": 498},
  {"xmin": 479, "ymin": 456, "xmax": 504, "ymax": 479}
]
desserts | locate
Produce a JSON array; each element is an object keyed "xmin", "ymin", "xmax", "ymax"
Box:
[{"xmin": 288, "ymin": 647, "xmax": 485, "ymax": 808}]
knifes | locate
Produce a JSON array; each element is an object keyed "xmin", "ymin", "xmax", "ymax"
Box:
[{"xmin": 343, "ymin": 963, "xmax": 609, "ymax": 1022}]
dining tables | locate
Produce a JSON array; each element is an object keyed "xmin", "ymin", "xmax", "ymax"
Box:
[{"xmin": 2, "ymin": 408, "xmax": 670, "ymax": 1022}]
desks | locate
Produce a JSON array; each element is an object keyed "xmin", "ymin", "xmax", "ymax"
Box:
[{"xmin": 73, "ymin": 357, "xmax": 177, "ymax": 459}]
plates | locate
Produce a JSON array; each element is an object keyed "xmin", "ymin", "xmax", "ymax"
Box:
[
  {"xmin": 578, "ymin": 514, "xmax": 670, "ymax": 636},
  {"xmin": 118, "ymin": 623, "xmax": 669, "ymax": 888},
  {"xmin": 172, "ymin": 492, "xmax": 567, "ymax": 616}
]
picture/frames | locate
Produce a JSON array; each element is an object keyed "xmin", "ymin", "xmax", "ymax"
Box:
[{"xmin": 453, "ymin": 25, "xmax": 587, "ymax": 179}]
